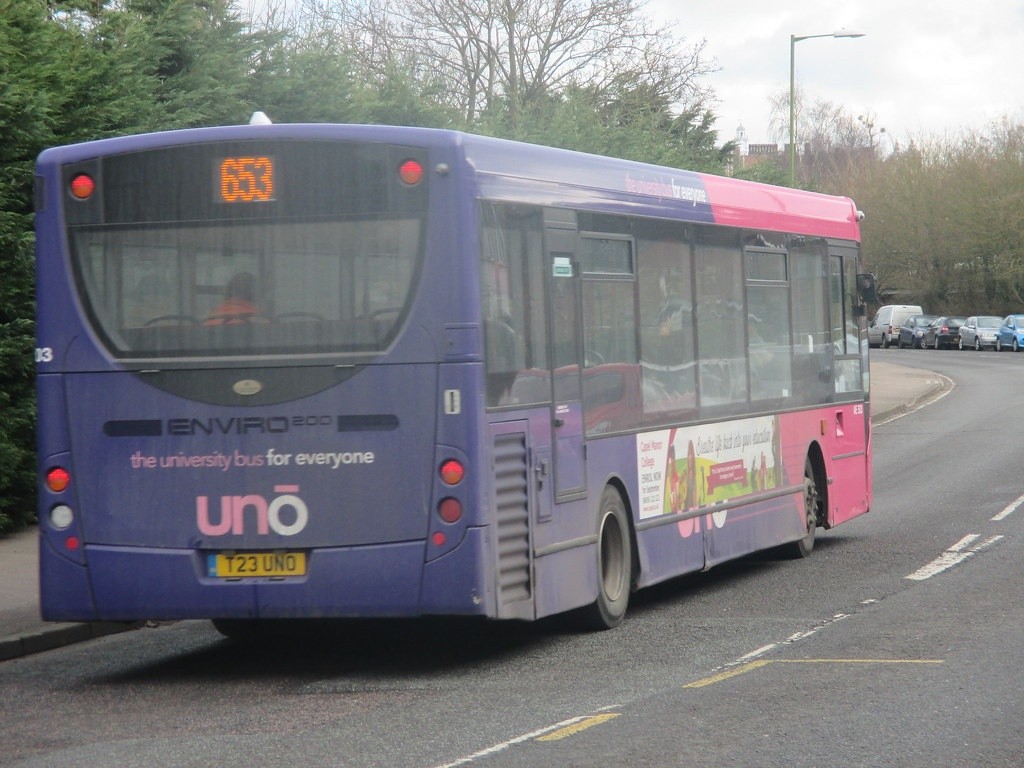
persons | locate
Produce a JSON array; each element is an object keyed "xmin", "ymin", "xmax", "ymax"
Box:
[
  {"xmin": 861, "ymin": 279, "xmax": 873, "ymax": 300},
  {"xmin": 658, "ymin": 252, "xmax": 694, "ymax": 366},
  {"xmin": 205, "ymin": 273, "xmax": 271, "ymax": 325}
]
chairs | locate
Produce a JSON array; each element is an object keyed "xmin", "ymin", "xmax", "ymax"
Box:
[{"xmin": 138, "ymin": 310, "xmax": 605, "ymax": 374}]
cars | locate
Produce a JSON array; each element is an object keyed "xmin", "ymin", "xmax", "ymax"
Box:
[
  {"xmin": 995, "ymin": 314, "xmax": 1024, "ymax": 351},
  {"xmin": 920, "ymin": 316, "xmax": 966, "ymax": 349},
  {"xmin": 957, "ymin": 316, "xmax": 1002, "ymax": 350},
  {"xmin": 897, "ymin": 315, "xmax": 936, "ymax": 349}
]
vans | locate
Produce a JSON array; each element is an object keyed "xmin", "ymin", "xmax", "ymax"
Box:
[{"xmin": 868, "ymin": 305, "xmax": 923, "ymax": 349}]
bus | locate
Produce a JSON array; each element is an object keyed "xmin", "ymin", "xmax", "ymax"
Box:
[{"xmin": 34, "ymin": 111, "xmax": 871, "ymax": 629}]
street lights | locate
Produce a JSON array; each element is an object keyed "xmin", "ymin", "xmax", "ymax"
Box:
[{"xmin": 787, "ymin": 32, "xmax": 869, "ymax": 180}]
